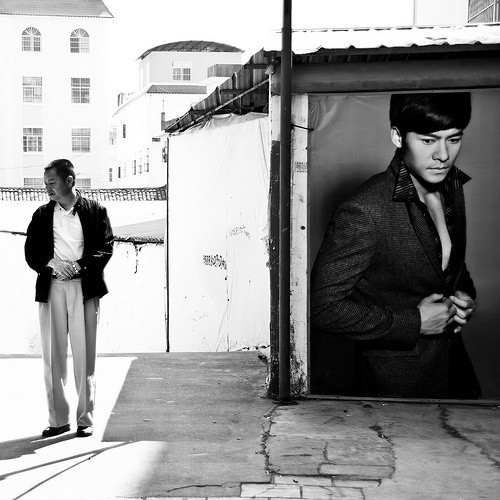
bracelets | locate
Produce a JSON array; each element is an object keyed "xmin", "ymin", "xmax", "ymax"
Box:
[{"xmin": 72, "ymin": 262, "xmax": 78, "ymax": 274}]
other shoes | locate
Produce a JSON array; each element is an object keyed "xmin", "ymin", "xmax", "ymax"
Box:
[
  {"xmin": 76, "ymin": 426, "xmax": 93, "ymax": 437},
  {"xmin": 43, "ymin": 424, "xmax": 70, "ymax": 438}
]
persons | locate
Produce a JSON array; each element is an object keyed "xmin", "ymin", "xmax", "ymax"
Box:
[
  {"xmin": 309, "ymin": 91, "xmax": 483, "ymax": 401},
  {"xmin": 24, "ymin": 158, "xmax": 114, "ymax": 437}
]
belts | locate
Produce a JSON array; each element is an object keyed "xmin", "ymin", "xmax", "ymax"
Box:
[{"xmin": 52, "ymin": 274, "xmax": 81, "ymax": 279}]
